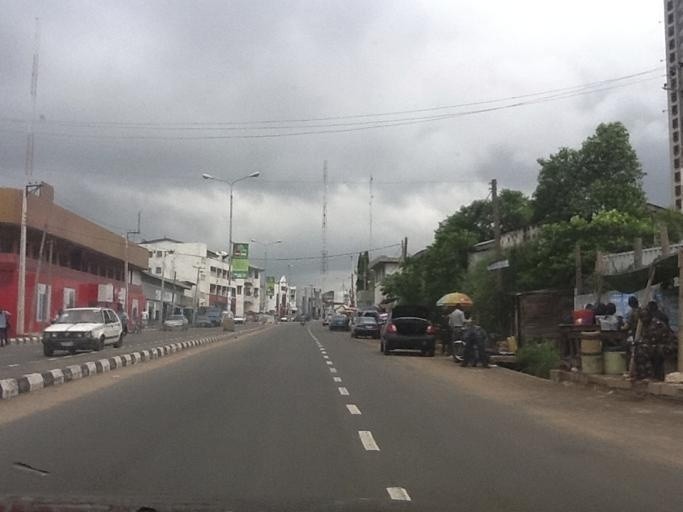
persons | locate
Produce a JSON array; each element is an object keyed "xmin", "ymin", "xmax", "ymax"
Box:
[
  {"xmin": 457, "ymin": 321, "xmax": 492, "ymax": 365},
  {"xmin": 448, "ymin": 301, "xmax": 472, "ymax": 357},
  {"xmin": 140, "ymin": 305, "xmax": 149, "ymax": 328},
  {"xmin": 561, "ymin": 294, "xmax": 678, "ymax": 381},
  {"xmin": 0, "ymin": 305, "xmax": 11, "ymax": 348},
  {"xmin": 121, "ymin": 307, "xmax": 129, "ymax": 336}
]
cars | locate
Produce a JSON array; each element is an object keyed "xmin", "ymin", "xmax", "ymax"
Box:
[
  {"xmin": 279, "ymin": 311, "xmax": 311, "ymax": 322},
  {"xmin": 163, "ymin": 314, "xmax": 188, "ymax": 332},
  {"xmin": 321, "ymin": 303, "xmax": 439, "ymax": 358},
  {"xmin": 42, "ymin": 306, "xmax": 124, "ymax": 358},
  {"xmin": 193, "ymin": 306, "xmax": 246, "ymax": 328}
]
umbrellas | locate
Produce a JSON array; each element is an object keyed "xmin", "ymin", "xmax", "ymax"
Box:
[{"xmin": 435, "ymin": 290, "xmax": 474, "ymax": 308}]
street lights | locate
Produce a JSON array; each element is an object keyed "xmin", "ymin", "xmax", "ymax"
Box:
[
  {"xmin": 199, "ymin": 171, "xmax": 261, "ymax": 332},
  {"xmin": 250, "ymin": 237, "xmax": 282, "ymax": 324}
]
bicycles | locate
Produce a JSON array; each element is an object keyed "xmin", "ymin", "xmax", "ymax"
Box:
[
  {"xmin": 124, "ymin": 316, "xmax": 146, "ymax": 334},
  {"xmin": 450, "ymin": 319, "xmax": 486, "ymax": 365}
]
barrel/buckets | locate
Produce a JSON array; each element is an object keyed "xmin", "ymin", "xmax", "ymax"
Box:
[
  {"xmin": 580, "ymin": 331, "xmax": 604, "ymax": 374},
  {"xmin": 603, "ymin": 350, "xmax": 628, "ymax": 375}
]
